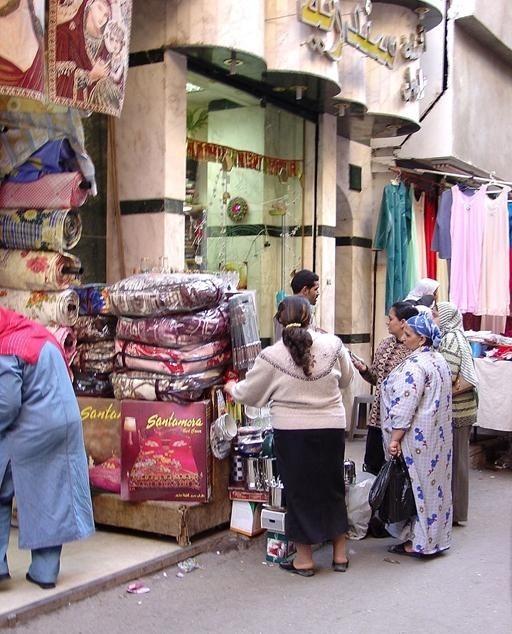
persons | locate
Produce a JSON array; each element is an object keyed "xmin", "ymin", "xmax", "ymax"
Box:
[
  {"xmin": 1, "ymin": 0, "xmax": 127, "ymax": 108},
  {"xmin": 273, "ymin": 268, "xmax": 320, "ymax": 339},
  {"xmin": 1, "ymin": 302, "xmax": 95, "ymax": 593},
  {"xmin": 348, "ymin": 275, "xmax": 481, "ymax": 561},
  {"xmin": 224, "ymin": 293, "xmax": 354, "ymax": 576}
]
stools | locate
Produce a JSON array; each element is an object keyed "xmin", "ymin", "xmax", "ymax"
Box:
[{"xmin": 347, "ymin": 394, "xmax": 375, "ymax": 440}]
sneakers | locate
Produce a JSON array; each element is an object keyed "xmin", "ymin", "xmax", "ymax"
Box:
[{"xmin": 26, "ymin": 573, "xmax": 55, "ymax": 588}]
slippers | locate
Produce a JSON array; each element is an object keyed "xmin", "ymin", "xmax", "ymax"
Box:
[
  {"xmin": 280, "ymin": 558, "xmax": 314, "ymax": 576},
  {"xmin": 387, "ymin": 541, "xmax": 424, "ymax": 558},
  {"xmin": 332, "ymin": 557, "xmax": 349, "ymax": 572}
]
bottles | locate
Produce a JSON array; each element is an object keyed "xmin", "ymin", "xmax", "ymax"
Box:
[{"xmin": 232, "ymin": 451, "xmax": 243, "ymax": 482}]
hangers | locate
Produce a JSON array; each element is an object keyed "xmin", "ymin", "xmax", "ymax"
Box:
[{"xmin": 387, "ymin": 166, "xmax": 512, "ymax": 206}]
